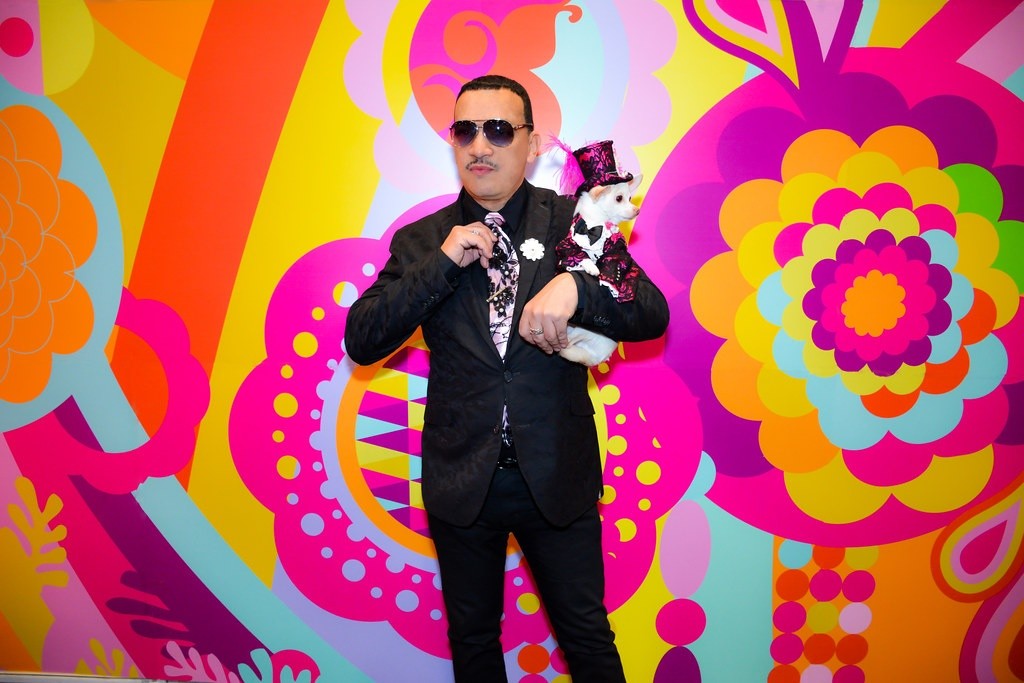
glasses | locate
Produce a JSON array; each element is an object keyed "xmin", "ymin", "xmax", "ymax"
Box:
[{"xmin": 449, "ymin": 118, "xmax": 534, "ymax": 148}]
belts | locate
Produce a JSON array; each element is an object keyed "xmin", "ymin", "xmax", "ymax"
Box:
[{"xmin": 497, "ymin": 459, "xmax": 517, "ymax": 470}]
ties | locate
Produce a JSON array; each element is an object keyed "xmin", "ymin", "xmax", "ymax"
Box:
[{"xmin": 484, "ymin": 212, "xmax": 520, "ymax": 446}]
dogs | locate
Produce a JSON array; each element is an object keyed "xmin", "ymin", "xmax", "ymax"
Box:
[{"xmin": 558, "ymin": 173, "xmax": 644, "ymax": 367}]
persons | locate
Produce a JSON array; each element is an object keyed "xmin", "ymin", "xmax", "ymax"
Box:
[{"xmin": 342, "ymin": 73, "xmax": 671, "ymax": 678}]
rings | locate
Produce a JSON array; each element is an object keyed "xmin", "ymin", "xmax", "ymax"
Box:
[
  {"xmin": 529, "ymin": 328, "xmax": 543, "ymax": 335},
  {"xmin": 472, "ymin": 229, "xmax": 479, "ymax": 236}
]
